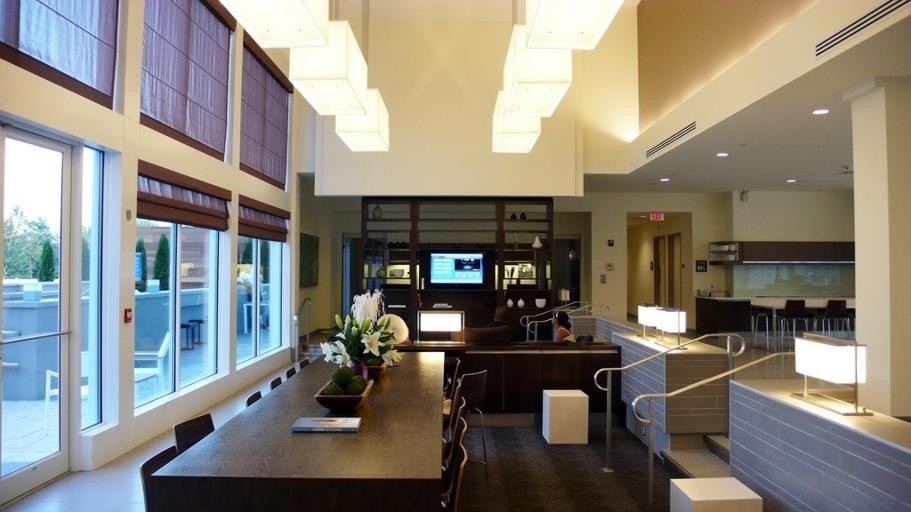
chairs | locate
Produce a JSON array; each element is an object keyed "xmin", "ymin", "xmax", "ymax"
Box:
[
  {"xmin": 442, "ymin": 378, "xmax": 461, "ymax": 444},
  {"xmin": 443, "ymin": 357, "xmax": 460, "ymax": 401},
  {"xmin": 271, "ymin": 376, "xmax": 282, "ymax": 390},
  {"xmin": 441, "ymin": 444, "xmax": 467, "ymax": 512},
  {"xmin": 813, "ymin": 299, "xmax": 850, "ymax": 339},
  {"xmin": 286, "ymin": 366, "xmax": 296, "ymax": 379},
  {"xmin": 299, "ymin": 358, "xmax": 309, "ymax": 369},
  {"xmin": 778, "ymin": 300, "xmax": 807, "ymax": 352},
  {"xmin": 442, "ymin": 396, "xmax": 465, "ymax": 471},
  {"xmin": 43, "ymin": 350, "xmax": 89, "ymax": 435},
  {"xmin": 173, "ymin": 412, "xmax": 215, "ymax": 455},
  {"xmin": 134, "ymin": 331, "xmax": 171, "ymax": 392},
  {"xmin": 139, "ymin": 445, "xmax": 178, "ymax": 512},
  {"xmin": 442, "ymin": 416, "xmax": 467, "ymax": 507},
  {"xmin": 461, "ymin": 369, "xmax": 488, "ymax": 465},
  {"xmin": 245, "ymin": 390, "xmax": 262, "ymax": 408}
]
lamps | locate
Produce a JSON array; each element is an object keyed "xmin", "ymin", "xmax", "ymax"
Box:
[
  {"xmin": 413, "ymin": 310, "xmax": 466, "ymax": 345},
  {"xmin": 790, "ymin": 331, "xmax": 874, "ymax": 416},
  {"xmin": 217, "ymin": 0, "xmax": 329, "ymax": 49},
  {"xmin": 637, "ymin": 303, "xmax": 658, "ymax": 341},
  {"xmin": 525, "ymin": 0, "xmax": 625, "ymax": 51},
  {"xmin": 334, "ymin": 0, "xmax": 390, "ymax": 153},
  {"xmin": 501, "ymin": 1, "xmax": 574, "ymax": 120},
  {"xmin": 288, "ymin": 0, "xmax": 368, "ymax": 117},
  {"xmin": 490, "ymin": 0, "xmax": 542, "ymax": 155},
  {"xmin": 655, "ymin": 306, "xmax": 688, "ymax": 350}
]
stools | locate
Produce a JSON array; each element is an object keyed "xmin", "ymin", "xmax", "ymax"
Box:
[
  {"xmin": 670, "ymin": 476, "xmax": 762, "ymax": 512},
  {"xmin": 180, "ymin": 323, "xmax": 197, "ymax": 350},
  {"xmin": 188, "ymin": 318, "xmax": 208, "ymax": 345},
  {"xmin": 542, "ymin": 389, "xmax": 589, "ymax": 444}
]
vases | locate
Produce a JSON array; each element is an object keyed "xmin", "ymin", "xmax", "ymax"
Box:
[
  {"xmin": 531, "ymin": 235, "xmax": 543, "ymax": 248},
  {"xmin": 349, "ymin": 361, "xmax": 368, "ymax": 378},
  {"xmin": 373, "ymin": 201, "xmax": 382, "ymax": 218},
  {"xmin": 517, "ymin": 298, "xmax": 524, "ymax": 309},
  {"xmin": 507, "ymin": 298, "xmax": 513, "ymax": 308}
]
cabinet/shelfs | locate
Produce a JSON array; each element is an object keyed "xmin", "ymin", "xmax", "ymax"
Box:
[
  {"xmin": 695, "ymin": 296, "xmax": 751, "ymax": 339},
  {"xmin": 708, "ymin": 241, "xmax": 855, "ymax": 265},
  {"xmin": 360, "ymin": 198, "xmax": 554, "ymax": 338},
  {"xmin": 504, "ymin": 355, "xmax": 578, "ymax": 414},
  {"xmin": 578, "ymin": 354, "xmax": 620, "ymax": 413},
  {"xmin": 461, "ymin": 354, "xmax": 501, "ymax": 414}
]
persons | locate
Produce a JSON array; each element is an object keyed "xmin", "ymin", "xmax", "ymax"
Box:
[
  {"xmin": 552, "ymin": 311, "xmax": 577, "ymax": 343},
  {"xmin": 239, "ymin": 261, "xmax": 267, "ymax": 330}
]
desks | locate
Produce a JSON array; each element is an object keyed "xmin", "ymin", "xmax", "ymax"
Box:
[
  {"xmin": 750, "ymin": 297, "xmax": 856, "ymax": 353},
  {"xmin": 149, "ymin": 351, "xmax": 445, "ymax": 498},
  {"xmin": 243, "ymin": 300, "xmax": 270, "ymax": 335}
]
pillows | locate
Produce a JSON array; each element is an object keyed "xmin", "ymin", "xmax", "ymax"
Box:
[{"xmin": 451, "ymin": 326, "xmax": 511, "ymax": 347}]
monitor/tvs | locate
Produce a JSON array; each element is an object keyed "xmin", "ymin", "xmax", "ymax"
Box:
[{"xmin": 424, "ymin": 247, "xmax": 495, "ymax": 289}]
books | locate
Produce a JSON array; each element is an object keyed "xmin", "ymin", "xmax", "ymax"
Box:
[{"xmin": 291, "ymin": 417, "xmax": 362, "ymax": 432}]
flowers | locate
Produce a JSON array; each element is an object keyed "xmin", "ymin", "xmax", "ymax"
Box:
[{"xmin": 320, "ymin": 314, "xmax": 402, "ymax": 366}]
